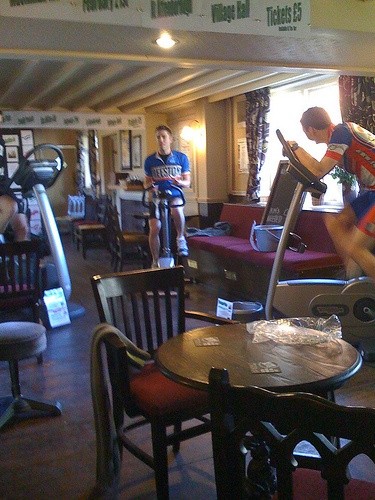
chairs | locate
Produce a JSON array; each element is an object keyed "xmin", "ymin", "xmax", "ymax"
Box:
[
  {"xmin": 55, "ymin": 194, "xmax": 85, "ymax": 234},
  {"xmin": 0, "ymin": 238, "xmax": 46, "ymax": 364},
  {"xmin": 208, "ymin": 366, "xmax": 375, "ymax": 500},
  {"xmin": 74, "ymin": 198, "xmax": 107, "ymax": 259},
  {"xmin": 91, "ymin": 265, "xmax": 212, "ymax": 500},
  {"xmin": 104, "ymin": 203, "xmax": 151, "ymax": 272}
]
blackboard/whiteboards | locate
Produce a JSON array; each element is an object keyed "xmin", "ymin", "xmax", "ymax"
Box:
[{"xmin": 260, "ymin": 160, "xmax": 307, "ymax": 233}]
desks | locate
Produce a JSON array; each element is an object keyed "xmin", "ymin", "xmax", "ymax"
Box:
[{"xmin": 155, "ymin": 322, "xmax": 362, "ymax": 485}]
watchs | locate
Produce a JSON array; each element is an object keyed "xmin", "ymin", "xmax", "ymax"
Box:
[{"xmin": 291, "ymin": 143, "xmax": 300, "ymax": 151}]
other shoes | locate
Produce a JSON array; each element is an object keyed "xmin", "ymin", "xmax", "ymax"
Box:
[{"xmin": 176, "ymin": 237, "xmax": 190, "ymax": 256}]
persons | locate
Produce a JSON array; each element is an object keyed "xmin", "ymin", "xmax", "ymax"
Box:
[
  {"xmin": 282, "ymin": 108, "xmax": 375, "ymax": 281},
  {"xmin": 0, "ymin": 110, "xmax": 30, "ymax": 259},
  {"xmin": 144, "ymin": 125, "xmax": 191, "ymax": 271}
]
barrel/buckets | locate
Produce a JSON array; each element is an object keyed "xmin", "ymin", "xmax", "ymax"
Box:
[
  {"xmin": 254, "ymin": 224, "xmax": 284, "ymax": 252},
  {"xmin": 232, "ymin": 301, "xmax": 263, "ymax": 323}
]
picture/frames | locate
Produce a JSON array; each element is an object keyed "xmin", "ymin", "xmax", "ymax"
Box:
[{"xmin": 120, "ymin": 130, "xmax": 132, "ymax": 170}]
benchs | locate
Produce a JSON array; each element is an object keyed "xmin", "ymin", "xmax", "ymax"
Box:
[{"xmin": 185, "ymin": 202, "xmax": 343, "ymax": 305}]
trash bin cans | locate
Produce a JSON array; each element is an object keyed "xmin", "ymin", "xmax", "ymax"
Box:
[{"xmin": 232, "ymin": 301, "xmax": 264, "ymax": 324}]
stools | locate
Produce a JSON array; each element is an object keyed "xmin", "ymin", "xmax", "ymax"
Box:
[{"xmin": 0, "ymin": 321, "xmax": 63, "ymax": 429}]
